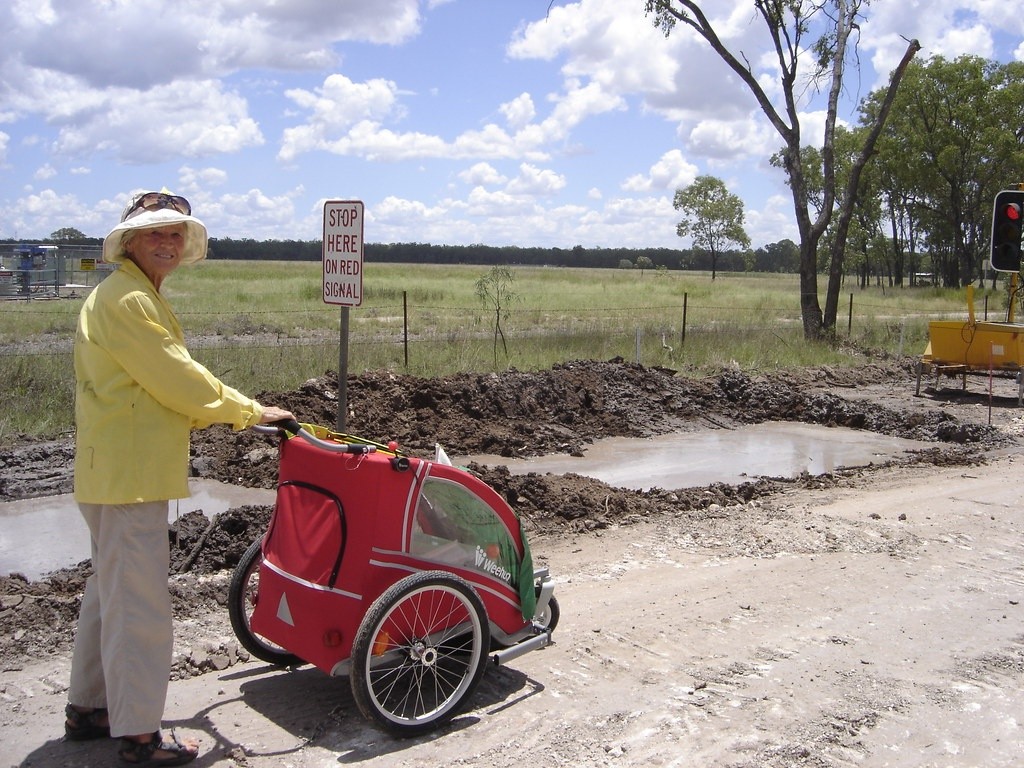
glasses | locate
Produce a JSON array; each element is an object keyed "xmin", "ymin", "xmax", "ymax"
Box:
[{"xmin": 124, "ymin": 192, "xmax": 191, "ymax": 216}]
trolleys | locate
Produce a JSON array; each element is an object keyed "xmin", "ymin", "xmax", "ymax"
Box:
[{"xmin": 225, "ymin": 402, "xmax": 561, "ymax": 735}]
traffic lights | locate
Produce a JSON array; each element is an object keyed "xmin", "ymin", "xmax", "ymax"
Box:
[{"xmin": 991, "ymin": 191, "xmax": 1024, "ymax": 273}]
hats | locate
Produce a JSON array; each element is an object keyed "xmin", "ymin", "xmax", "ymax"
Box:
[{"xmin": 102, "ymin": 187, "xmax": 208, "ymax": 264}]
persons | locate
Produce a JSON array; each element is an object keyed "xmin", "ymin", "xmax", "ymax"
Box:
[{"xmin": 66, "ymin": 186, "xmax": 297, "ymax": 768}]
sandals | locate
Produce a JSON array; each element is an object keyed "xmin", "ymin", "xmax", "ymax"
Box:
[
  {"xmin": 64, "ymin": 704, "xmax": 113, "ymax": 738},
  {"xmin": 116, "ymin": 731, "xmax": 199, "ymax": 768}
]
